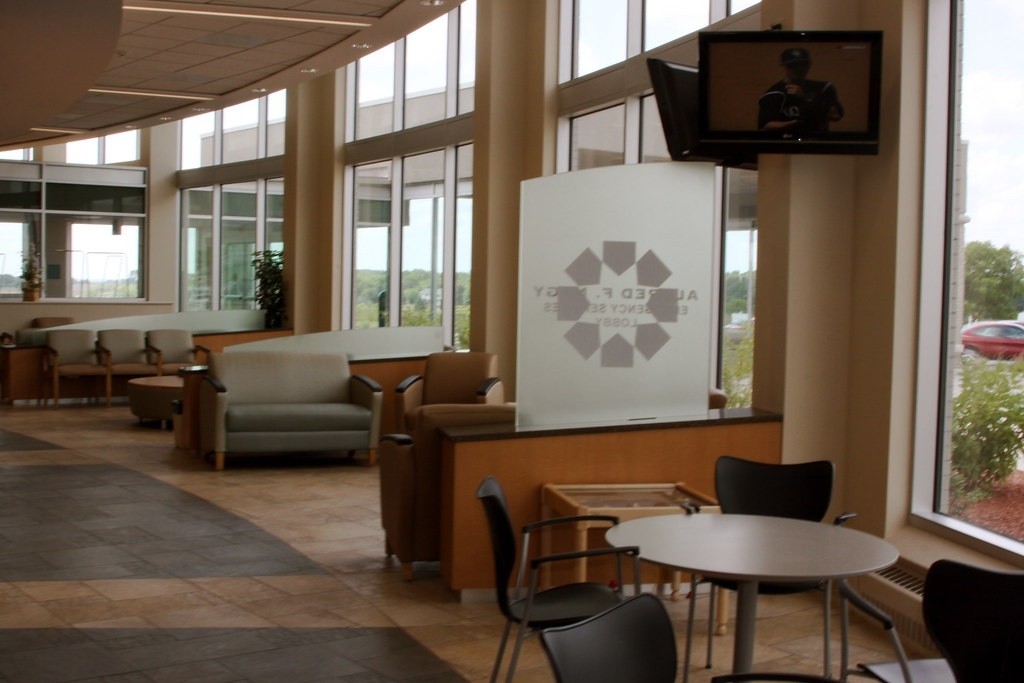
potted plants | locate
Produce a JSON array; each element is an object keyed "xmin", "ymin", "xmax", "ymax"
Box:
[
  {"xmin": 251, "ymin": 247, "xmax": 289, "ymax": 330},
  {"xmin": 20, "ymin": 243, "xmax": 42, "ymax": 301}
]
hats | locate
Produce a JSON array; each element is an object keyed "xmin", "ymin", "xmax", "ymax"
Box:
[{"xmin": 781, "ymin": 48, "xmax": 809, "ymax": 65}]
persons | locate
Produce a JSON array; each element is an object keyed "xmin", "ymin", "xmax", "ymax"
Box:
[{"xmin": 757, "ymin": 47, "xmax": 844, "ymax": 135}]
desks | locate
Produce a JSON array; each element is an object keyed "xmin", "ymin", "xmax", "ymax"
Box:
[
  {"xmin": 128, "ymin": 376, "xmax": 185, "ymax": 431},
  {"xmin": 540, "ymin": 482, "xmax": 729, "ymax": 635},
  {"xmin": 604, "ymin": 514, "xmax": 899, "ymax": 675}
]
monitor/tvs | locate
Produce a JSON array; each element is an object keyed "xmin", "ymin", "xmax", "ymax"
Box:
[{"xmin": 646, "ymin": 31, "xmax": 884, "ymax": 171}]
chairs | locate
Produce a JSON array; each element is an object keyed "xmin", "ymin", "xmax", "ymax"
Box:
[
  {"xmin": 696, "ymin": 455, "xmax": 835, "ymax": 678},
  {"xmin": 839, "ymin": 560, "xmax": 1024, "ymax": 683},
  {"xmin": 475, "ymin": 474, "xmax": 641, "ymax": 683},
  {"xmin": 39, "ymin": 329, "xmax": 210, "ymax": 406},
  {"xmin": 538, "ymin": 592, "xmax": 840, "ymax": 683}
]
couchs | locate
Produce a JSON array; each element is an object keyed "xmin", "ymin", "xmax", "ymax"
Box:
[
  {"xmin": 379, "ymin": 390, "xmax": 728, "ymax": 581},
  {"xmin": 201, "ymin": 351, "xmax": 383, "ymax": 472},
  {"xmin": 394, "ymin": 349, "xmax": 502, "ymax": 434}
]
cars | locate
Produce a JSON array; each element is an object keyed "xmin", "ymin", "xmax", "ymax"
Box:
[{"xmin": 960, "ymin": 321, "xmax": 1024, "ymax": 359}]
